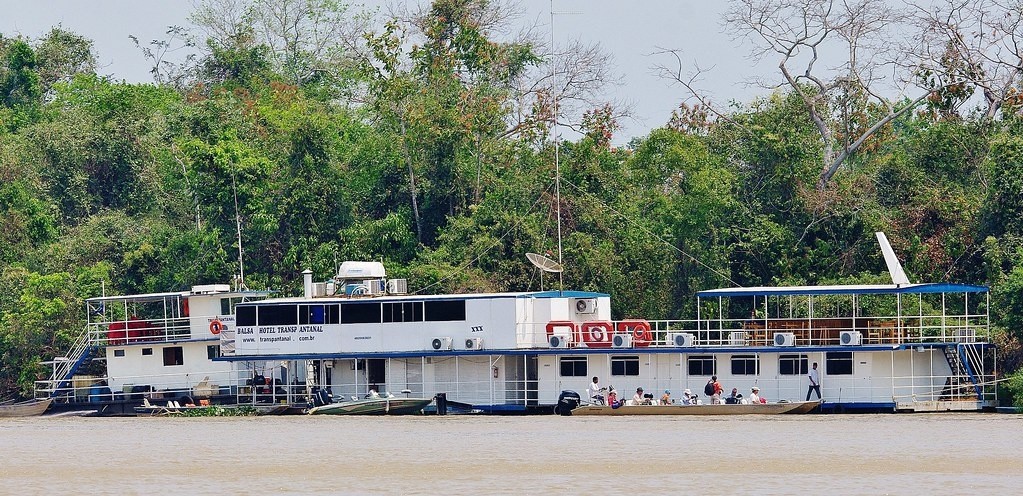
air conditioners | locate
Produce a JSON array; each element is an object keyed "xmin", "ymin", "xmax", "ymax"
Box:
[
  {"xmin": 773, "ymin": 332, "xmax": 795, "ymax": 346},
  {"xmin": 667, "ymin": 333, "xmax": 687, "ymax": 345},
  {"xmin": 311, "ymin": 283, "xmax": 335, "ymax": 296},
  {"xmin": 955, "ymin": 329, "xmax": 975, "ymax": 343},
  {"xmin": 464, "ymin": 337, "xmax": 481, "ymax": 350},
  {"xmin": 612, "ymin": 334, "xmax": 632, "ymax": 348},
  {"xmin": 548, "ymin": 335, "xmax": 569, "ymax": 348},
  {"xmin": 362, "ymin": 280, "xmax": 381, "ymax": 295},
  {"xmin": 674, "ymin": 333, "xmax": 694, "ymax": 347},
  {"xmin": 389, "ymin": 279, "xmax": 407, "ymax": 293},
  {"xmin": 731, "ymin": 332, "xmax": 750, "ymax": 346},
  {"xmin": 431, "ymin": 337, "xmax": 453, "ymax": 349},
  {"xmin": 840, "ymin": 331, "xmax": 860, "ymax": 345},
  {"xmin": 574, "ymin": 298, "xmax": 597, "ymax": 314}
]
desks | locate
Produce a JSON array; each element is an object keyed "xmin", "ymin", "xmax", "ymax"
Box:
[{"xmin": 877, "ymin": 322, "xmax": 914, "ymax": 343}]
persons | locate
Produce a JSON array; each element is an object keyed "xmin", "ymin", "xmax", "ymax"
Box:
[
  {"xmin": 806, "ymin": 363, "xmax": 821, "ymax": 401},
  {"xmin": 590, "ymin": 377, "xmax": 604, "ymax": 405},
  {"xmin": 608, "ymin": 375, "xmax": 763, "ymax": 405}
]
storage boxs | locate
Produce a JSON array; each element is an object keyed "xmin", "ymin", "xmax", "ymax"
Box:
[
  {"xmin": 72, "ymin": 375, "xmax": 103, "ymax": 395},
  {"xmin": 193, "ymin": 377, "xmax": 219, "ymax": 396}
]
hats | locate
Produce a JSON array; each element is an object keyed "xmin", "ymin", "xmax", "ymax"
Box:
[
  {"xmin": 683, "ymin": 389, "xmax": 692, "ymax": 395},
  {"xmin": 751, "ymin": 387, "xmax": 760, "ymax": 391},
  {"xmin": 636, "ymin": 387, "xmax": 644, "ymax": 392},
  {"xmin": 609, "ymin": 389, "xmax": 617, "ymax": 395},
  {"xmin": 665, "ymin": 390, "xmax": 670, "ymax": 394}
]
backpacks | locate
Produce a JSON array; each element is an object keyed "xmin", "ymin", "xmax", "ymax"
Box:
[{"xmin": 705, "ymin": 381, "xmax": 717, "ymax": 396}]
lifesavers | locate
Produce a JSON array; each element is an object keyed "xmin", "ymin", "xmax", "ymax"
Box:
[
  {"xmin": 591, "ymin": 328, "xmax": 603, "ymax": 341},
  {"xmin": 210, "ymin": 321, "xmax": 222, "ymax": 335},
  {"xmin": 634, "ymin": 325, "xmax": 647, "ymax": 338}
]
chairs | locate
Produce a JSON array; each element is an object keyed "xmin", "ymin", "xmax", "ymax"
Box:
[
  {"xmin": 868, "ymin": 320, "xmax": 921, "ymax": 344},
  {"xmin": 744, "ymin": 320, "xmax": 846, "ymax": 344}
]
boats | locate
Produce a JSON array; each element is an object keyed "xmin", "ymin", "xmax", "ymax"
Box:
[
  {"xmin": 554, "ymin": 390, "xmax": 823, "ymax": 415},
  {"xmin": 308, "ymin": 388, "xmax": 433, "ymax": 415},
  {"xmin": 31, "ymin": 225, "xmax": 999, "ymax": 413},
  {"xmin": 434, "ymin": 390, "xmax": 580, "ymax": 416}
]
